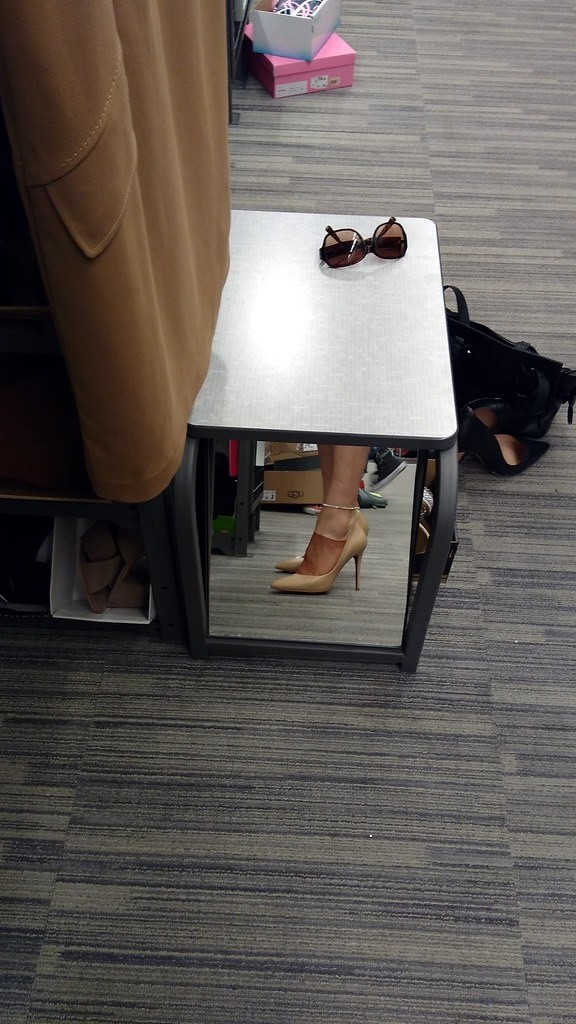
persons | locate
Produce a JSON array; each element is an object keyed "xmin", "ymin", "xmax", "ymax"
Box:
[
  {"xmin": 367, "ymin": 445, "xmax": 406, "ymax": 491},
  {"xmin": 270, "ymin": 443, "xmax": 373, "ymax": 594}
]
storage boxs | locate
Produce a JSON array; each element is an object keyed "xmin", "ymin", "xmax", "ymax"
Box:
[
  {"xmin": 252, "ymin": 0, "xmax": 342, "ymax": 62},
  {"xmin": 242, "ymin": 23, "xmax": 356, "ymax": 98},
  {"xmin": 48, "ymin": 515, "xmax": 156, "ymax": 625},
  {"xmin": 261, "ymin": 469, "xmax": 323, "ymax": 506}
]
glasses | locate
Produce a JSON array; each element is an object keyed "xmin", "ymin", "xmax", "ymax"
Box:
[{"xmin": 319, "ymin": 216, "xmax": 407, "ymax": 268}]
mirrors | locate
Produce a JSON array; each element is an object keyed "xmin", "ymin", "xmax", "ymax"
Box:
[{"xmin": 196, "ymin": 431, "xmax": 426, "ymax": 664}]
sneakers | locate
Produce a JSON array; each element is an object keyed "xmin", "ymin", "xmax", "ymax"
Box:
[{"xmin": 370, "ymin": 452, "xmax": 407, "ymax": 490}]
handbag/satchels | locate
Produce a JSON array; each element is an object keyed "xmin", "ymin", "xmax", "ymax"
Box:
[{"xmin": 443, "ymin": 285, "xmax": 576, "ymax": 439}]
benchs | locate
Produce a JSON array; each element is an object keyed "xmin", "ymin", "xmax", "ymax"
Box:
[{"xmin": 177, "ymin": 209, "xmax": 460, "ymax": 674}]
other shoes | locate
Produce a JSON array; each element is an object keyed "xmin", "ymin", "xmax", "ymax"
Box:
[
  {"xmin": 304, "ymin": 504, "xmax": 323, "ymax": 515},
  {"xmin": 274, "ymin": 0, "xmax": 321, "ymax": 19}
]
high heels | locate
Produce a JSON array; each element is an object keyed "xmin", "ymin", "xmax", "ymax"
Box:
[
  {"xmin": 275, "ymin": 510, "xmax": 369, "ymax": 572},
  {"xmin": 271, "ymin": 521, "xmax": 368, "ymax": 593},
  {"xmin": 462, "ymin": 397, "xmax": 551, "ymax": 477},
  {"xmin": 78, "ymin": 516, "xmax": 150, "ymax": 614}
]
